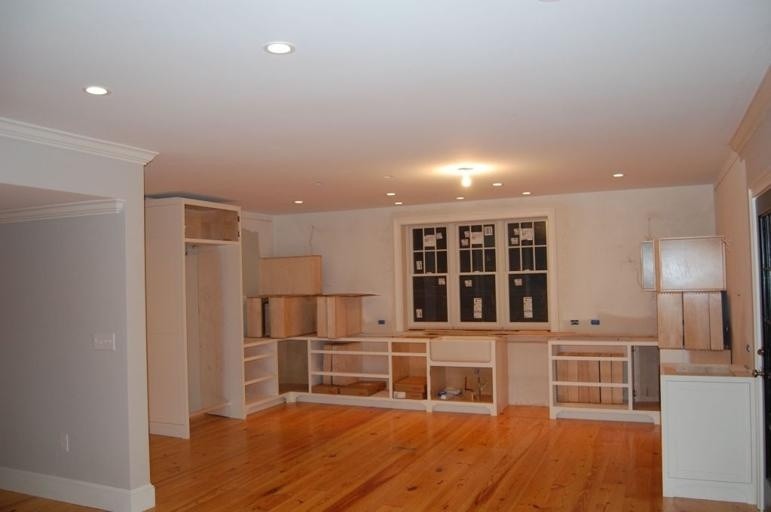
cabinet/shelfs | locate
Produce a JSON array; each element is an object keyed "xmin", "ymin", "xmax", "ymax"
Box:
[
  {"xmin": 660, "ymin": 363, "xmax": 757, "ymax": 501},
  {"xmin": 243, "ymin": 340, "xmax": 278, "ymax": 419},
  {"xmin": 145, "ymin": 196, "xmax": 246, "ymax": 440},
  {"xmin": 428, "ymin": 337, "xmax": 509, "ymax": 416},
  {"xmin": 307, "ymin": 339, "xmax": 390, "ymax": 410},
  {"xmin": 548, "ymin": 341, "xmax": 661, "ymax": 425},
  {"xmin": 390, "ymin": 341, "xmax": 428, "ymax": 411}
]
